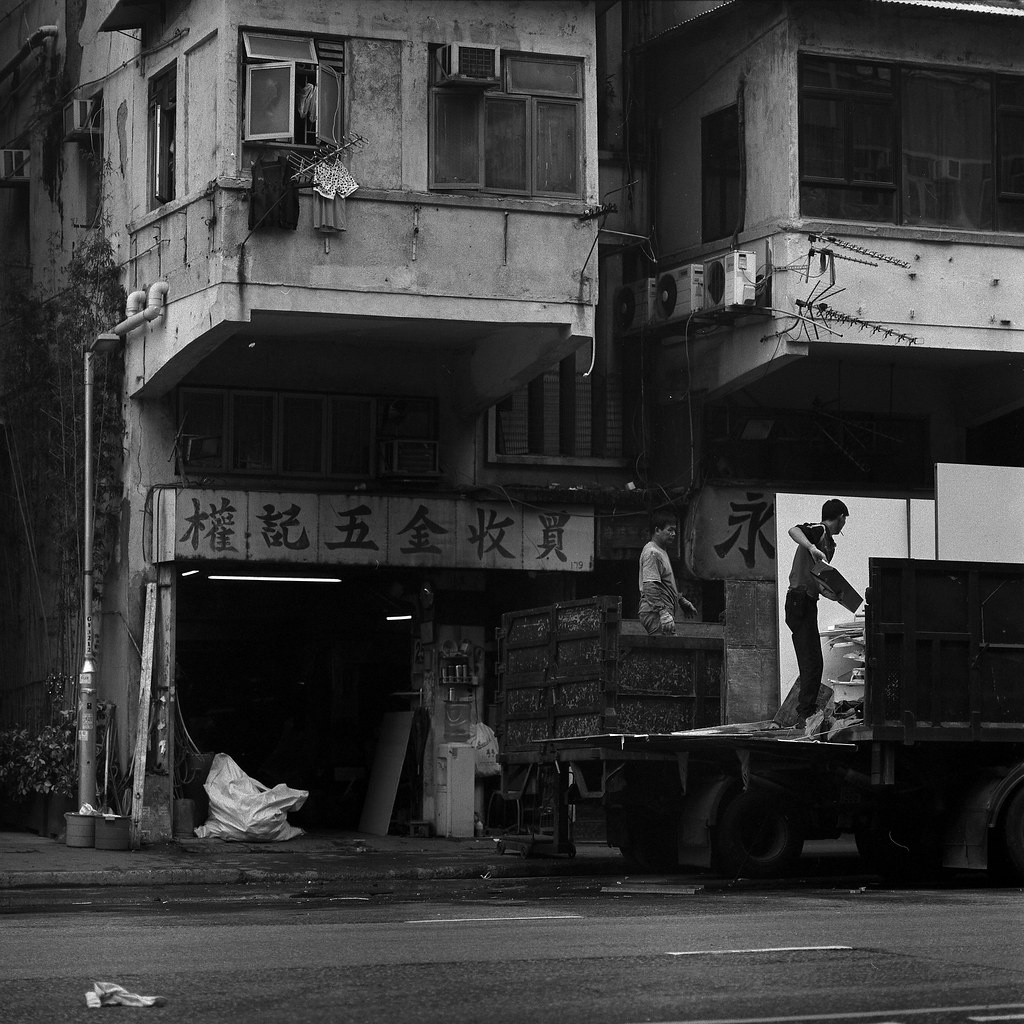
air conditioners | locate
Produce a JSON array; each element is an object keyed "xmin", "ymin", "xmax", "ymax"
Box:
[
  {"xmin": 654, "ymin": 264, "xmax": 704, "ymax": 323},
  {"xmin": 63, "ymin": 99, "xmax": 102, "ymax": 141},
  {"xmin": 702, "ymin": 249, "xmax": 757, "ymax": 307},
  {"xmin": 614, "ymin": 278, "xmax": 656, "ymax": 332},
  {"xmin": 0, "ymin": 149, "xmax": 32, "ymax": 183},
  {"xmin": 378, "ymin": 435, "xmax": 439, "ymax": 478},
  {"xmin": 436, "ymin": 40, "xmax": 501, "ymax": 91}
]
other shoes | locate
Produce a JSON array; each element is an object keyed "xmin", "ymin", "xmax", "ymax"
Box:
[{"xmin": 795, "ymin": 715, "xmax": 806, "ymax": 728}]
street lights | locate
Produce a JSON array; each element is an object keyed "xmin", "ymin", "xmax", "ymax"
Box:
[{"xmin": 77, "ymin": 333, "xmax": 120, "ymax": 810}]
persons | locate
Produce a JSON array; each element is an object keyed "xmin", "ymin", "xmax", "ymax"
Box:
[
  {"xmin": 638, "ymin": 512, "xmax": 699, "ymax": 635},
  {"xmin": 786, "ymin": 499, "xmax": 852, "ymax": 729}
]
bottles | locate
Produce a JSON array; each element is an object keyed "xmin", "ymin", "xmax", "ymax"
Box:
[{"xmin": 444, "ymin": 703, "xmax": 472, "ymax": 742}]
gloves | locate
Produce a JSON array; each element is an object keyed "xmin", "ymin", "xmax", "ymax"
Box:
[
  {"xmin": 658, "ymin": 610, "xmax": 676, "ymax": 633},
  {"xmin": 678, "ymin": 597, "xmax": 698, "ymax": 620},
  {"xmin": 809, "ymin": 544, "xmax": 829, "ymax": 564}
]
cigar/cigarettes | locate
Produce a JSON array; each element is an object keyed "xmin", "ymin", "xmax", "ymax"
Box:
[{"xmin": 840, "ymin": 530, "xmax": 844, "ymax": 536}]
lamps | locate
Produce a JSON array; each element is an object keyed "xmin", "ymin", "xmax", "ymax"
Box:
[{"xmin": 168, "ymin": 408, "xmax": 222, "ymax": 487}]
